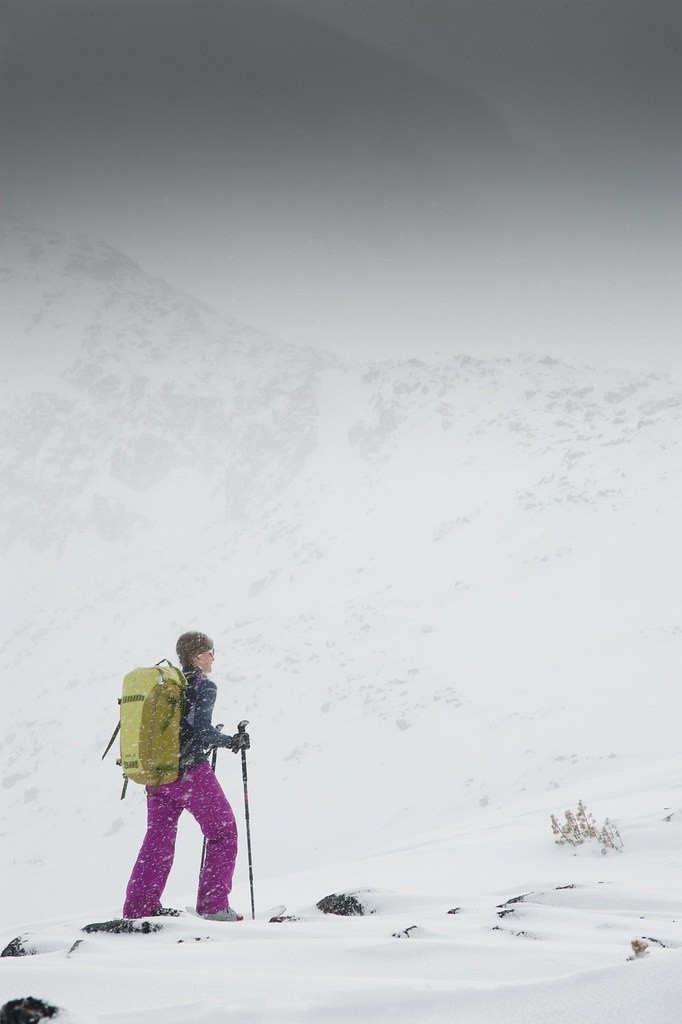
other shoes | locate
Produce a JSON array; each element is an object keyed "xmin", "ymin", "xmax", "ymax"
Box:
[
  {"xmin": 153, "ymin": 908, "xmax": 179, "ymax": 918},
  {"xmin": 197, "ymin": 906, "xmax": 243, "ymax": 921}
]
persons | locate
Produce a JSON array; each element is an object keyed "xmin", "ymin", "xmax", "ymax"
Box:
[{"xmin": 124, "ymin": 630, "xmax": 250, "ymax": 920}]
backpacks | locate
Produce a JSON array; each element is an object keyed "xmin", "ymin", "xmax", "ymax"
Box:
[{"xmin": 115, "ymin": 659, "xmax": 188, "ymax": 785}]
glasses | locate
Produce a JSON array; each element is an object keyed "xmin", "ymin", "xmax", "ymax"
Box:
[{"xmin": 197, "ymin": 649, "xmax": 215, "ymax": 658}]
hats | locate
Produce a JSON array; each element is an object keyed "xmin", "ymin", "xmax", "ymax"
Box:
[{"xmin": 176, "ymin": 631, "xmax": 214, "ymax": 660}]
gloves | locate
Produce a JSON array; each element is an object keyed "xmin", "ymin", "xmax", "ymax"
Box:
[{"xmin": 227, "ymin": 733, "xmax": 250, "ymax": 754}]
